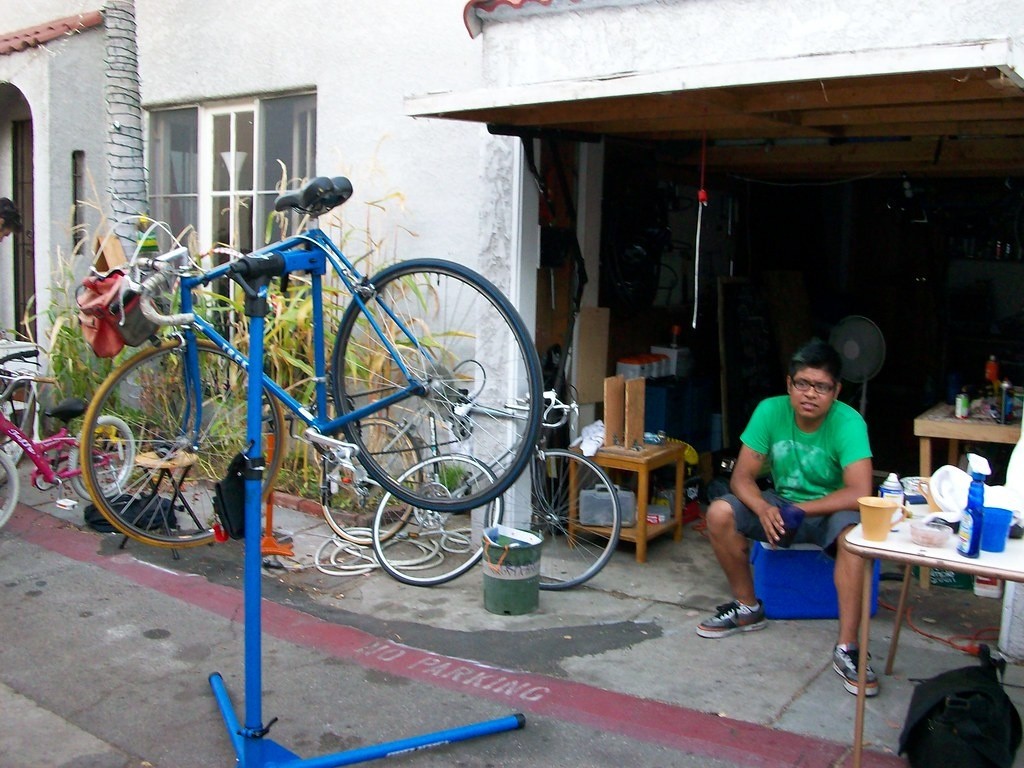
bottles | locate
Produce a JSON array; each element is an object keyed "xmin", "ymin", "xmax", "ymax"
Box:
[
  {"xmin": 878, "ymin": 473, "xmax": 904, "ymax": 532},
  {"xmin": 985, "ymin": 355, "xmax": 997, "ymax": 384},
  {"xmin": 667, "ymin": 325, "xmax": 681, "ymax": 349},
  {"xmin": 998, "ymin": 380, "xmax": 1014, "ymax": 424}
]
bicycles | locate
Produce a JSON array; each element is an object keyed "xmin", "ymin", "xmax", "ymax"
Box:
[
  {"xmin": 317, "ymin": 384, "xmax": 622, "ymax": 591},
  {"xmin": 79, "ymin": 175, "xmax": 544, "ymax": 549},
  {"xmin": 0, "ymin": 349, "xmax": 135, "ymax": 530},
  {"xmin": 622, "ymin": 487, "xmax": 623, "ymax": 488}
]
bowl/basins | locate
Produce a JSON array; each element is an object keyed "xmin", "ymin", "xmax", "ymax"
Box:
[{"xmin": 910, "ymin": 523, "xmax": 953, "ymax": 547}]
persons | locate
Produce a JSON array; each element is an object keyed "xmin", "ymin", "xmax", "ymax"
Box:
[{"xmin": 696, "ymin": 341, "xmax": 880, "ymax": 697}]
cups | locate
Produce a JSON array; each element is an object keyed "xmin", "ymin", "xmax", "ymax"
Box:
[
  {"xmin": 979, "ymin": 506, "xmax": 1013, "ymax": 552},
  {"xmin": 774, "ymin": 504, "xmax": 807, "ymax": 548},
  {"xmin": 856, "ymin": 496, "xmax": 908, "ymax": 542}
]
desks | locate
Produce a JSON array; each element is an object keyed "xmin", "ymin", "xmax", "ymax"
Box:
[
  {"xmin": 842, "ymin": 517, "xmax": 1024, "ymax": 768},
  {"xmin": 913, "ymin": 403, "xmax": 1021, "ymax": 590},
  {"xmin": 568, "ymin": 441, "xmax": 687, "ymax": 564}
]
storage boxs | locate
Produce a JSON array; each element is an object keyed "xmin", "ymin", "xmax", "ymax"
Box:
[
  {"xmin": 749, "ymin": 540, "xmax": 882, "ymax": 619},
  {"xmin": 710, "ymin": 414, "xmax": 736, "ymax": 452}
]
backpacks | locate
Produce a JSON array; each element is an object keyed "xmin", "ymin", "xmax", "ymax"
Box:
[{"xmin": 84, "ymin": 493, "xmax": 177, "ymax": 533}]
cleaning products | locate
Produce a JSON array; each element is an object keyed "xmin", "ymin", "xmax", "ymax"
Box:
[
  {"xmin": 955, "ymin": 453, "xmax": 991, "ymax": 561},
  {"xmin": 985, "ymin": 355, "xmax": 997, "ymax": 384}
]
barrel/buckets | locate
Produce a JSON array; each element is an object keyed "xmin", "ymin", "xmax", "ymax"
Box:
[{"xmin": 481, "ymin": 528, "xmax": 544, "ymax": 615}]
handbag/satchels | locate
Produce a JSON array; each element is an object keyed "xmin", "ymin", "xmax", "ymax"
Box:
[
  {"xmin": 212, "ymin": 452, "xmax": 245, "ymax": 541},
  {"xmin": 897, "ymin": 654, "xmax": 1022, "ymax": 768}
]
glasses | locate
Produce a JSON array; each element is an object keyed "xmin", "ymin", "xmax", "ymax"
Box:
[{"xmin": 790, "ymin": 375, "xmax": 835, "ymax": 394}]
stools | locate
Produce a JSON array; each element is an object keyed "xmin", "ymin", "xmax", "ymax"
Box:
[{"xmin": 119, "ymin": 452, "xmax": 215, "ymax": 560}]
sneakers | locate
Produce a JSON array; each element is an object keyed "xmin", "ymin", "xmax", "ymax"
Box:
[
  {"xmin": 832, "ymin": 643, "xmax": 879, "ymax": 696},
  {"xmin": 696, "ymin": 599, "xmax": 768, "ymax": 638}
]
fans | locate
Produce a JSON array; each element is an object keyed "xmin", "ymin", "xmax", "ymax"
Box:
[{"xmin": 828, "ymin": 315, "xmax": 901, "ymax": 479}]
